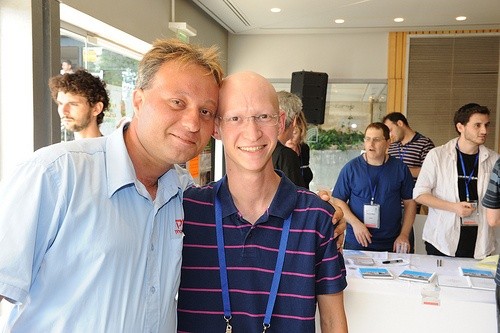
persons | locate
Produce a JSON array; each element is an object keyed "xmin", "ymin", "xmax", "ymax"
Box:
[
  {"xmin": 0, "ymin": 41, "xmax": 346, "ymax": 333},
  {"xmin": 482, "ymin": 158, "xmax": 500, "ymax": 333},
  {"xmin": 384, "ymin": 112, "xmax": 435, "ymax": 254},
  {"xmin": 60, "ymin": 60, "xmax": 74, "ymax": 74},
  {"xmin": 49, "ymin": 71, "xmax": 109, "ymax": 138},
  {"xmin": 285, "ymin": 112, "xmax": 313, "ymax": 190},
  {"xmin": 330, "ymin": 122, "xmax": 416, "ymax": 254},
  {"xmin": 176, "ymin": 71, "xmax": 348, "ymax": 333},
  {"xmin": 272, "ymin": 91, "xmax": 302, "ymax": 185},
  {"xmin": 413, "ymin": 103, "xmax": 500, "ymax": 260}
]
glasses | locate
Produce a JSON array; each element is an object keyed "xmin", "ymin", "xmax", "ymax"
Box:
[
  {"xmin": 362, "ymin": 136, "xmax": 386, "ymax": 142},
  {"xmin": 217, "ymin": 112, "xmax": 281, "ymax": 127}
]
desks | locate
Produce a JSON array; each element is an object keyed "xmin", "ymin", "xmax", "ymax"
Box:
[{"xmin": 316, "ymin": 248, "xmax": 500, "ymax": 333}]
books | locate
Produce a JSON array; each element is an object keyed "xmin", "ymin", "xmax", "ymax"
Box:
[
  {"xmin": 399, "ymin": 269, "xmax": 436, "ymax": 282},
  {"xmin": 380, "ymin": 258, "xmax": 408, "ymax": 267},
  {"xmin": 358, "ymin": 266, "xmax": 394, "ymax": 279},
  {"xmin": 345, "ymin": 256, "xmax": 375, "ymax": 269},
  {"xmin": 438, "ymin": 275, "xmax": 496, "ymax": 290},
  {"xmin": 373, "ymin": 252, "xmax": 389, "ymax": 259},
  {"xmin": 459, "ymin": 266, "xmax": 494, "ymax": 278}
]
